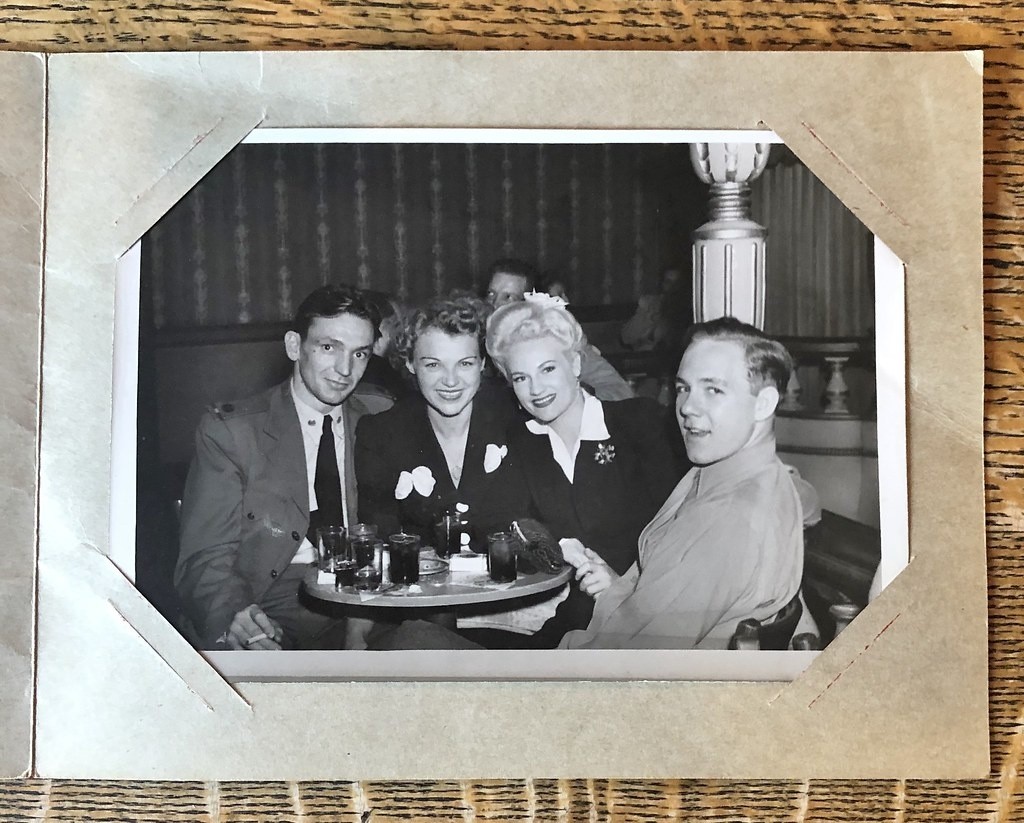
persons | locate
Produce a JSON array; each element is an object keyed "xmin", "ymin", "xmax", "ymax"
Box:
[
  {"xmin": 557, "ymin": 315, "xmax": 805, "ymax": 650},
  {"xmin": 345, "ymin": 288, "xmax": 592, "ymax": 649},
  {"xmin": 172, "ymin": 285, "xmax": 372, "ymax": 652},
  {"xmin": 621, "ymin": 267, "xmax": 693, "ymax": 407},
  {"xmin": 487, "ymin": 259, "xmax": 637, "ymax": 401},
  {"xmin": 483, "ymin": 294, "xmax": 694, "ymax": 649},
  {"xmin": 360, "ymin": 290, "xmax": 415, "ymax": 398}
]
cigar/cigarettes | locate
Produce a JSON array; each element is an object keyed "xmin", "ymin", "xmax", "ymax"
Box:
[{"xmin": 246, "ymin": 634, "xmax": 268, "ymax": 643}]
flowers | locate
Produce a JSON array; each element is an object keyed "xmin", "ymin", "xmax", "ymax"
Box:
[
  {"xmin": 523, "ymin": 288, "xmax": 569, "ymax": 311},
  {"xmin": 483, "ymin": 444, "xmax": 508, "ymax": 472},
  {"xmin": 594, "ymin": 444, "xmax": 617, "ymax": 466},
  {"xmin": 394, "ymin": 466, "xmax": 436, "ymax": 499},
  {"xmin": 372, "ymin": 315, "xmax": 394, "ymax": 356}
]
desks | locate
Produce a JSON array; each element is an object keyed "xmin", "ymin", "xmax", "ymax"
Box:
[{"xmin": 302, "ymin": 555, "xmax": 575, "ymax": 633}]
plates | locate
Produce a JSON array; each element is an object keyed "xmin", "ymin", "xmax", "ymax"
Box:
[{"xmin": 418, "ymin": 557, "xmax": 449, "ymax": 577}]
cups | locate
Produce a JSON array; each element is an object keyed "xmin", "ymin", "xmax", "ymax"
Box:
[
  {"xmin": 488, "ymin": 532, "xmax": 519, "ymax": 586},
  {"xmin": 348, "ymin": 523, "xmax": 384, "ymax": 592},
  {"xmin": 388, "ymin": 533, "xmax": 421, "ymax": 586},
  {"xmin": 316, "ymin": 525, "xmax": 345, "ymax": 573},
  {"xmin": 432, "ymin": 510, "xmax": 462, "ymax": 560}
]
